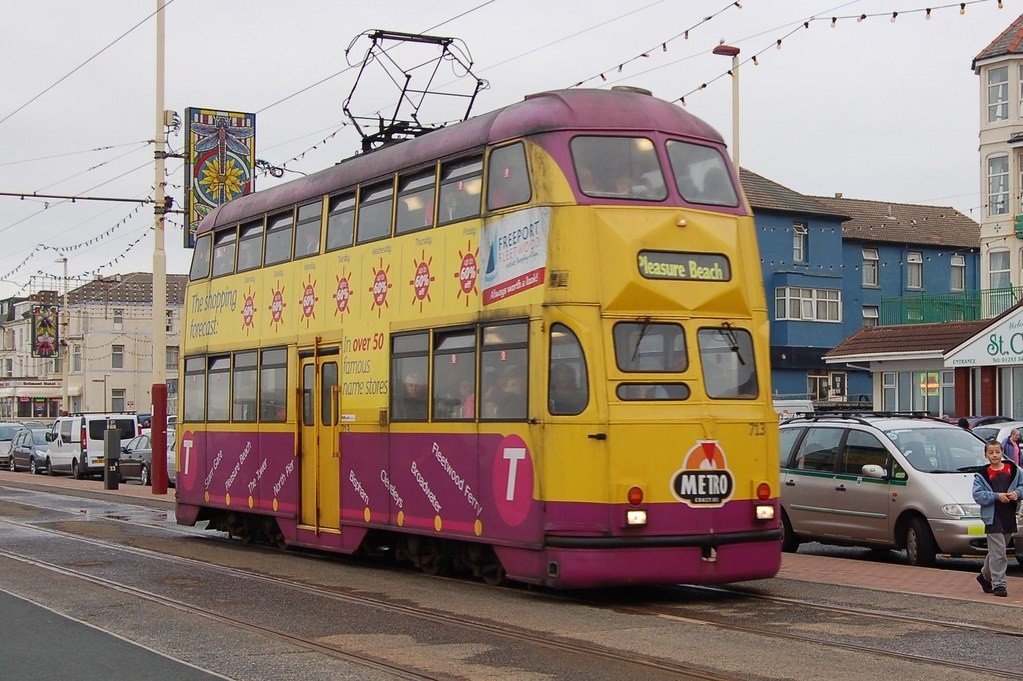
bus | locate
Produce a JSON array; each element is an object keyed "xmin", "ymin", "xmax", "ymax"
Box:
[{"xmin": 173, "ymin": 29, "xmax": 785, "ymax": 590}]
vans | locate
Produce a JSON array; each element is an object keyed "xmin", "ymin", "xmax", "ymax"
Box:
[
  {"xmin": 45, "ymin": 411, "xmax": 140, "ymax": 480},
  {"xmin": 771, "ymin": 399, "xmax": 815, "ymax": 467}
]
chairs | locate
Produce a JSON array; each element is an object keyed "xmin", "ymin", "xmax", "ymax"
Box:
[
  {"xmin": 191, "ymin": 396, "xmax": 556, "ymax": 420},
  {"xmin": 903, "ymin": 441, "xmax": 935, "ymax": 470},
  {"xmin": 219, "ymin": 197, "xmax": 457, "ymax": 274}
]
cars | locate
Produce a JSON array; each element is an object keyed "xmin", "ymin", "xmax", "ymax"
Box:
[
  {"xmin": 0, "ymin": 420, "xmax": 28, "ymax": 468},
  {"xmin": 137, "ymin": 412, "xmax": 177, "ymax": 434},
  {"xmin": 118, "ymin": 434, "xmax": 152, "ymax": 485},
  {"xmin": 910, "ymin": 416, "xmax": 1019, "ymax": 448},
  {"xmin": 21, "ymin": 420, "xmax": 49, "ymax": 428},
  {"xmin": 971, "ymin": 421, "xmax": 1023, "ymax": 456},
  {"xmin": 166, "ymin": 435, "xmax": 176, "ymax": 487},
  {"xmin": 9, "ymin": 427, "xmax": 53, "ymax": 474}
]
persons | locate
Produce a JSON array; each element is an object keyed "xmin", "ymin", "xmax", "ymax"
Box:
[
  {"xmin": 425, "ymin": 184, "xmax": 456, "ymax": 225},
  {"xmin": 958, "ymin": 418, "xmax": 969, "ymax": 429},
  {"xmin": 399, "ymin": 371, "xmax": 425, "ymax": 419},
  {"xmin": 972, "ymin": 440, "xmax": 1023, "ymax": 597},
  {"xmin": 551, "ymin": 364, "xmax": 577, "ymax": 413},
  {"xmin": 883, "ymin": 437, "xmax": 925, "ymax": 479},
  {"xmin": 582, "ymin": 149, "xmax": 632, "ymax": 194},
  {"xmin": 437, "ymin": 379, "xmax": 471, "ymax": 418},
  {"xmin": 142, "ymin": 420, "xmax": 150, "ymax": 428},
  {"xmin": 465, "ymin": 372, "xmax": 500, "ymax": 417},
  {"xmin": 942, "ymin": 415, "xmax": 949, "ymax": 421},
  {"xmin": 1001, "ymin": 428, "xmax": 1023, "ymax": 469},
  {"xmin": 496, "ymin": 372, "xmax": 527, "ymax": 417},
  {"xmin": 680, "ymin": 347, "xmax": 713, "ymax": 398}
]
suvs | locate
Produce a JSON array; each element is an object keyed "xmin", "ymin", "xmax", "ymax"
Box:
[{"xmin": 775, "ymin": 401, "xmax": 1023, "ymax": 567}]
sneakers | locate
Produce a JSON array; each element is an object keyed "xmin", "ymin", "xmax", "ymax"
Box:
[{"xmin": 976, "ymin": 574, "xmax": 1009, "ymax": 596}]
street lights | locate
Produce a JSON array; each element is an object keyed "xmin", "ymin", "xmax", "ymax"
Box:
[
  {"xmin": 709, "ymin": 46, "xmax": 743, "ymax": 176},
  {"xmin": 102, "ymin": 373, "xmax": 112, "ymax": 411},
  {"xmin": 53, "ymin": 256, "xmax": 70, "ymax": 416}
]
